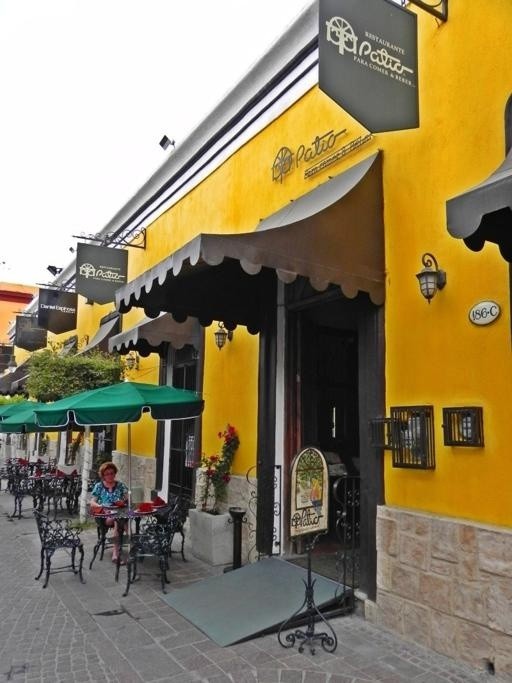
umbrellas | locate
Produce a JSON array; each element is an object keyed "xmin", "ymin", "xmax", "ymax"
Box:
[
  {"xmin": 0, "ymin": 399, "xmax": 49, "ymax": 421},
  {"xmin": 0, "ymin": 403, "xmax": 104, "ymax": 457},
  {"xmin": 34, "ymin": 379, "xmax": 205, "ymax": 553}
]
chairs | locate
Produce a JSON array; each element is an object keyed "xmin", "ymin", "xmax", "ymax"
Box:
[{"xmin": 0, "ymin": 457, "xmax": 193, "ymax": 597}]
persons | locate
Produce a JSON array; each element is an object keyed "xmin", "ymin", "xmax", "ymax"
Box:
[
  {"xmin": 310, "ymin": 474, "xmax": 322, "ymax": 502},
  {"xmin": 89, "ymin": 461, "xmax": 133, "ymax": 565}
]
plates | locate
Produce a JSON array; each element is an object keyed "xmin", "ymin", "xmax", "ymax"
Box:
[
  {"xmin": 151, "ymin": 505, "xmax": 165, "ymax": 508},
  {"xmin": 105, "ymin": 506, "xmax": 119, "ymax": 509},
  {"xmin": 133, "ymin": 508, "xmax": 158, "ymax": 514},
  {"xmin": 94, "ymin": 510, "xmax": 117, "ymax": 516}
]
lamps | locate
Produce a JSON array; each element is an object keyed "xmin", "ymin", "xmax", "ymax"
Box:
[
  {"xmin": 415, "ymin": 252, "xmax": 446, "ymax": 304},
  {"xmin": 126, "ymin": 351, "xmax": 139, "ymax": 370},
  {"xmin": 214, "ymin": 320, "xmax": 233, "ymax": 351}
]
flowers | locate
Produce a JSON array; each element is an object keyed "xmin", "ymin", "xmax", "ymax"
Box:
[{"xmin": 197, "ymin": 423, "xmax": 240, "ymax": 514}]
